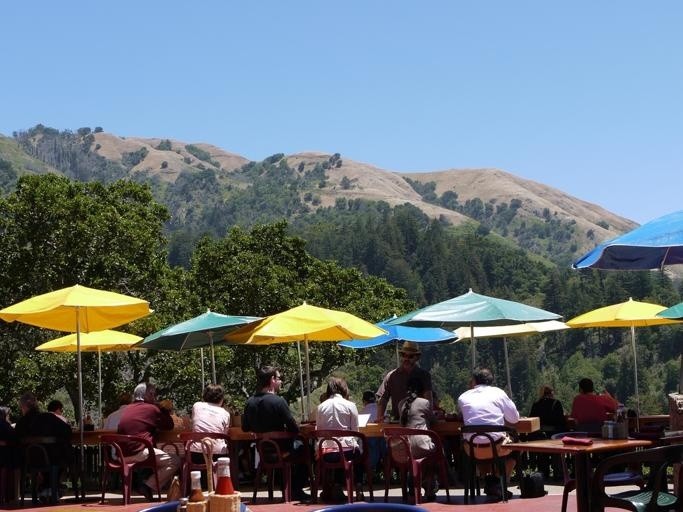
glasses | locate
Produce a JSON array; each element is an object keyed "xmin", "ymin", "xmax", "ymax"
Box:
[
  {"xmin": 400, "ymin": 353, "xmax": 418, "ymax": 360},
  {"xmin": 60, "ymin": 408, "xmax": 64, "ymax": 411},
  {"xmin": 275, "ymin": 376, "xmax": 284, "ymax": 380}
]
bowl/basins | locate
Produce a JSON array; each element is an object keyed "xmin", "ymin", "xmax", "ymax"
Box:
[
  {"xmin": 137, "ymin": 495, "xmax": 252, "ymax": 512},
  {"xmin": 310, "ymin": 502, "xmax": 429, "ymax": 512}
]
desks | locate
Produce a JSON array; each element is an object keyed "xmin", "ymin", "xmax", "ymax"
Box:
[
  {"xmin": 501, "ymin": 437, "xmax": 652, "ymax": 512},
  {"xmin": 16, "ymin": 424, "xmax": 541, "ymax": 504}
]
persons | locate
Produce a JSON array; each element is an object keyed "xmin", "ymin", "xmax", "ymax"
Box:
[
  {"xmin": 308, "ymin": 376, "xmax": 363, "ymax": 505},
  {"xmin": 155, "ymin": 399, "xmax": 186, "ymax": 458},
  {"xmin": 0, "ymin": 407, "xmax": 15, "ymax": 442},
  {"xmin": 358, "ymin": 390, "xmax": 389, "ymax": 423},
  {"xmin": 46, "ymin": 399, "xmax": 68, "ymax": 423},
  {"xmin": 241, "ymin": 365, "xmax": 316, "ymax": 504},
  {"xmin": 115, "ymin": 382, "xmax": 178, "ymax": 502},
  {"xmin": 185, "ymin": 383, "xmax": 231, "ymax": 491},
  {"xmin": 15, "ymin": 392, "xmax": 72, "ymax": 504},
  {"xmin": 570, "ymin": 378, "xmax": 619, "ymax": 439},
  {"xmin": 374, "ymin": 341, "xmax": 434, "ymax": 424},
  {"xmin": 528, "ymin": 385, "xmax": 564, "ymax": 477},
  {"xmin": 457, "ymin": 368, "xmax": 520, "ymax": 500},
  {"xmin": 311, "ymin": 393, "xmax": 329, "ymax": 422},
  {"xmin": 103, "ymin": 389, "xmax": 131, "ymax": 464},
  {"xmin": 396, "ymin": 377, "xmax": 437, "ymax": 504}
]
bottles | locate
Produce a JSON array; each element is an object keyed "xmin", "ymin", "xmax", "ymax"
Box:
[
  {"xmin": 168, "ymin": 476, "xmax": 181, "ymax": 501},
  {"xmin": 602, "ymin": 421, "xmax": 607, "ymax": 438},
  {"xmin": 216, "ymin": 458, "xmax": 233, "ymax": 494},
  {"xmin": 607, "ymin": 421, "xmax": 613, "ymax": 439},
  {"xmin": 189, "ymin": 471, "xmax": 205, "ymax": 501},
  {"xmin": 241, "ymin": 502, "xmax": 252, "ymax": 512}
]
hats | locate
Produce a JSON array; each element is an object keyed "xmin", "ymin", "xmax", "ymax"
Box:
[
  {"xmin": 544, "ymin": 386, "xmax": 555, "ymax": 394},
  {"xmin": 364, "ymin": 391, "xmax": 376, "ymax": 402},
  {"xmin": 159, "ymin": 400, "xmax": 173, "ymax": 411},
  {"xmin": 397, "ymin": 340, "xmax": 422, "ymax": 355}
]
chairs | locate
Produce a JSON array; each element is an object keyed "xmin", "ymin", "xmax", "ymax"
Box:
[
  {"xmin": 552, "ymin": 433, "xmax": 644, "ymax": 512},
  {"xmin": 462, "ymin": 426, "xmax": 525, "ymax": 502},
  {"xmin": 593, "ymin": 443, "xmax": 683, "ymax": 512},
  {"xmin": 181, "ymin": 432, "xmax": 239, "ymax": 496},
  {"xmin": 628, "ymin": 415, "xmax": 671, "ymax": 439},
  {"xmin": 20, "ymin": 434, "xmax": 88, "ymax": 505},
  {"xmin": 99, "ymin": 435, "xmax": 162, "ymax": 505},
  {"xmin": 250, "ymin": 432, "xmax": 315, "ymax": 503},
  {"xmin": 383, "ymin": 427, "xmax": 452, "ymax": 505},
  {"xmin": 313, "ymin": 429, "xmax": 376, "ymax": 503}
]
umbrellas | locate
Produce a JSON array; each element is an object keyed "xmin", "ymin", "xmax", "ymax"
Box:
[
  {"xmin": 222, "ymin": 300, "xmax": 389, "ymax": 422},
  {"xmin": 447, "ymin": 321, "xmax": 571, "ymax": 400},
  {"xmin": 655, "ymin": 302, "xmax": 683, "ymax": 321},
  {"xmin": 570, "ymin": 208, "xmax": 683, "ymax": 271},
  {"xmin": 34, "ymin": 329, "xmax": 144, "ymax": 429},
  {"xmin": 0, "ymin": 284, "xmax": 155, "ymax": 469},
  {"xmin": 384, "ymin": 288, "xmax": 564, "ymax": 368},
  {"xmin": 564, "ymin": 296, "xmax": 683, "ymax": 433},
  {"xmin": 130, "ymin": 308, "xmax": 267, "ymax": 386},
  {"xmin": 336, "ymin": 313, "xmax": 459, "ymax": 369}
]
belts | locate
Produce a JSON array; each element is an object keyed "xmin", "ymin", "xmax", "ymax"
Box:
[
  {"xmin": 116, "ymin": 448, "xmax": 145, "ymax": 457},
  {"xmin": 464, "ymin": 437, "xmax": 507, "ymax": 448}
]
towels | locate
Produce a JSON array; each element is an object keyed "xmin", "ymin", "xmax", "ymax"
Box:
[
  {"xmin": 562, "ymin": 436, "xmax": 593, "ymax": 446},
  {"xmin": 630, "ymin": 431, "xmax": 660, "ymax": 439}
]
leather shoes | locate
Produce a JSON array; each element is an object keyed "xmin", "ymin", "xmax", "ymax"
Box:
[
  {"xmin": 552, "ymin": 473, "xmax": 563, "ymax": 481},
  {"xmin": 499, "ymin": 490, "xmax": 513, "ymax": 499},
  {"xmin": 425, "ymin": 491, "xmax": 436, "ymax": 501},
  {"xmin": 282, "ymin": 493, "xmax": 295, "ymax": 501},
  {"xmin": 485, "ymin": 487, "xmax": 490, "ymax": 492},
  {"xmin": 356, "ymin": 487, "xmax": 364, "ymax": 499},
  {"xmin": 300, "ymin": 491, "xmax": 311, "ymax": 499},
  {"xmin": 408, "ymin": 490, "xmax": 418, "ymax": 497}
]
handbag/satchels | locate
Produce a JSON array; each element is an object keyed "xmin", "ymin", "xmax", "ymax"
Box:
[
  {"xmin": 168, "ymin": 475, "xmax": 183, "ymax": 503},
  {"xmin": 385, "ymin": 432, "xmax": 411, "ymax": 463},
  {"xmin": 519, "ymin": 471, "xmax": 545, "ymax": 498},
  {"xmin": 320, "ymin": 485, "xmax": 346, "ymax": 503}
]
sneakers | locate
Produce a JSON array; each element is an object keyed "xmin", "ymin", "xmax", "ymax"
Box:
[
  {"xmin": 48, "ymin": 488, "xmax": 53, "ymax": 503},
  {"xmin": 245, "ymin": 475, "xmax": 256, "ymax": 479},
  {"xmin": 40, "ymin": 490, "xmax": 49, "ymax": 504},
  {"xmin": 238, "ymin": 478, "xmax": 252, "ymax": 486},
  {"xmin": 138, "ymin": 483, "xmax": 154, "ymax": 502}
]
